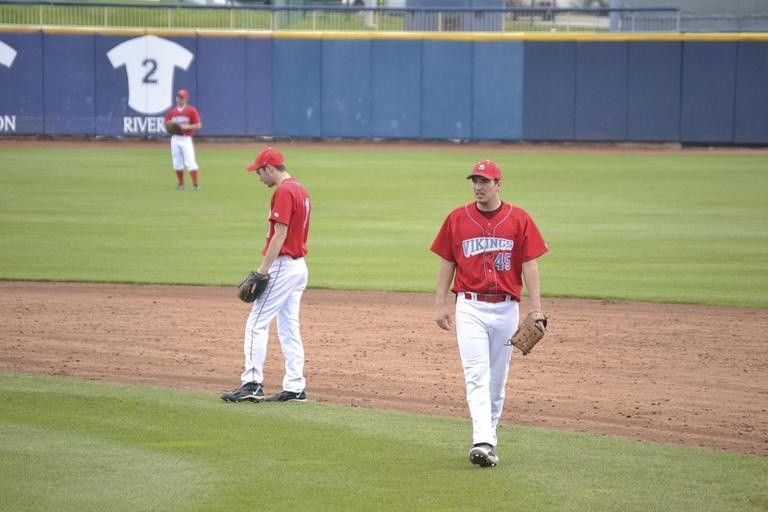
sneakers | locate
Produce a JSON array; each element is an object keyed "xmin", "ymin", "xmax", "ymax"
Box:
[
  {"xmin": 221, "ymin": 383, "xmax": 264, "ymax": 403},
  {"xmin": 177, "ymin": 182, "xmax": 200, "ymax": 191},
  {"xmin": 468, "ymin": 443, "xmax": 499, "ymax": 468},
  {"xmin": 266, "ymin": 389, "xmax": 307, "ymax": 402}
]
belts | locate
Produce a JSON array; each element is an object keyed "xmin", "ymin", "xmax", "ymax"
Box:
[{"xmin": 464, "ymin": 291, "xmax": 514, "ymax": 305}]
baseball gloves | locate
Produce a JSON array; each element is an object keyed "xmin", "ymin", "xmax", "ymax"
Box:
[
  {"xmin": 166, "ymin": 123, "xmax": 180, "ymax": 135},
  {"xmin": 511, "ymin": 311, "xmax": 548, "ymax": 356},
  {"xmin": 236, "ymin": 271, "xmax": 271, "ymax": 302}
]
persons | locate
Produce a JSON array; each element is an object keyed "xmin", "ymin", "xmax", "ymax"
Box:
[
  {"xmin": 165, "ymin": 89, "xmax": 202, "ymax": 190},
  {"xmin": 218, "ymin": 149, "xmax": 311, "ymax": 403},
  {"xmin": 430, "ymin": 159, "xmax": 548, "ymax": 468}
]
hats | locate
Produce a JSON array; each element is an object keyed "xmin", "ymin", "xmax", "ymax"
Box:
[
  {"xmin": 243, "ymin": 147, "xmax": 285, "ymax": 171},
  {"xmin": 176, "ymin": 89, "xmax": 189, "ymax": 99},
  {"xmin": 466, "ymin": 160, "xmax": 503, "ymax": 181}
]
